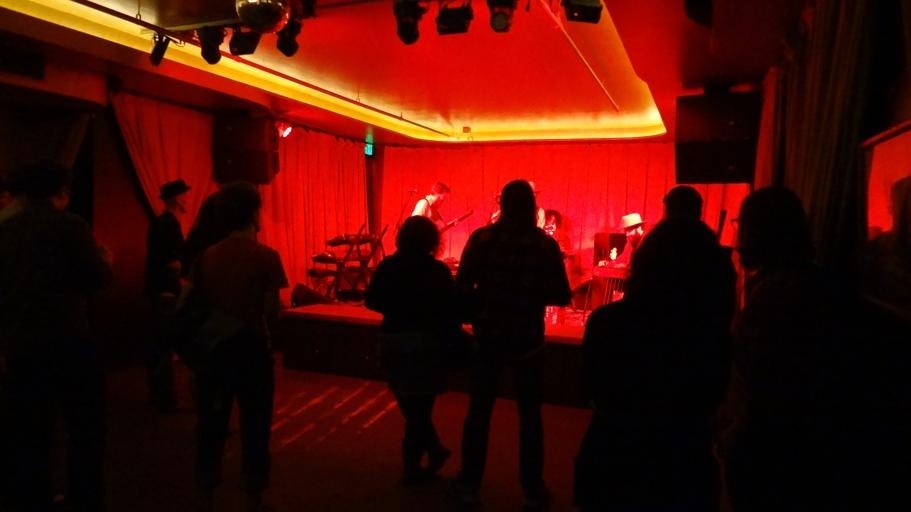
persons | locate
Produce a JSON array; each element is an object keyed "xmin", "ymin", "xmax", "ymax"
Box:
[
  {"xmin": 161, "ymin": 183, "xmax": 283, "ymax": 492},
  {"xmin": 441, "ymin": 184, "xmax": 571, "ymax": 511},
  {"xmin": 576, "ymin": 233, "xmax": 728, "ymax": 512},
  {"xmin": 1, "ymin": 158, "xmax": 114, "ymax": 511},
  {"xmin": 616, "ymin": 213, "xmax": 648, "ymax": 262},
  {"xmin": 183, "ymin": 196, "xmax": 232, "ymax": 439},
  {"xmin": 406, "ymin": 177, "xmax": 449, "ymax": 216},
  {"xmin": 656, "ymin": 186, "xmax": 735, "ymax": 402},
  {"xmin": 142, "ymin": 181, "xmax": 189, "ymax": 412},
  {"xmin": 365, "ymin": 214, "xmax": 460, "ymax": 485},
  {"xmin": 490, "ymin": 180, "xmax": 546, "ymax": 231},
  {"xmin": 718, "ymin": 186, "xmax": 847, "ymax": 511},
  {"xmin": 544, "ymin": 210, "xmax": 574, "ymax": 268}
]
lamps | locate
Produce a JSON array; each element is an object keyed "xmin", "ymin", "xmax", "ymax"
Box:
[
  {"xmin": 148, "ymin": 35, "xmax": 169, "ymax": 64},
  {"xmin": 228, "ymin": 24, "xmax": 261, "ymax": 57},
  {"xmin": 490, "ymin": 11, "xmax": 509, "ymax": 31},
  {"xmin": 563, "ymin": 1, "xmax": 602, "ymax": 25},
  {"xmin": 277, "ymin": 18, "xmax": 300, "ymax": 57},
  {"xmin": 436, "ymin": 5, "xmax": 474, "ymax": 35},
  {"xmin": 196, "ymin": 25, "xmax": 224, "ymax": 64},
  {"xmin": 393, "ymin": 2, "xmax": 426, "ymax": 44}
]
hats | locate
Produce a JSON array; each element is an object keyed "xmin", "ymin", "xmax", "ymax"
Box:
[
  {"xmin": 160, "ymin": 179, "xmax": 192, "ymax": 200},
  {"xmin": 616, "ymin": 211, "xmax": 647, "ymax": 230}
]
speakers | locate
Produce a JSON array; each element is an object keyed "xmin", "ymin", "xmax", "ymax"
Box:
[{"xmin": 674, "ymin": 94, "xmax": 763, "ymax": 183}]
